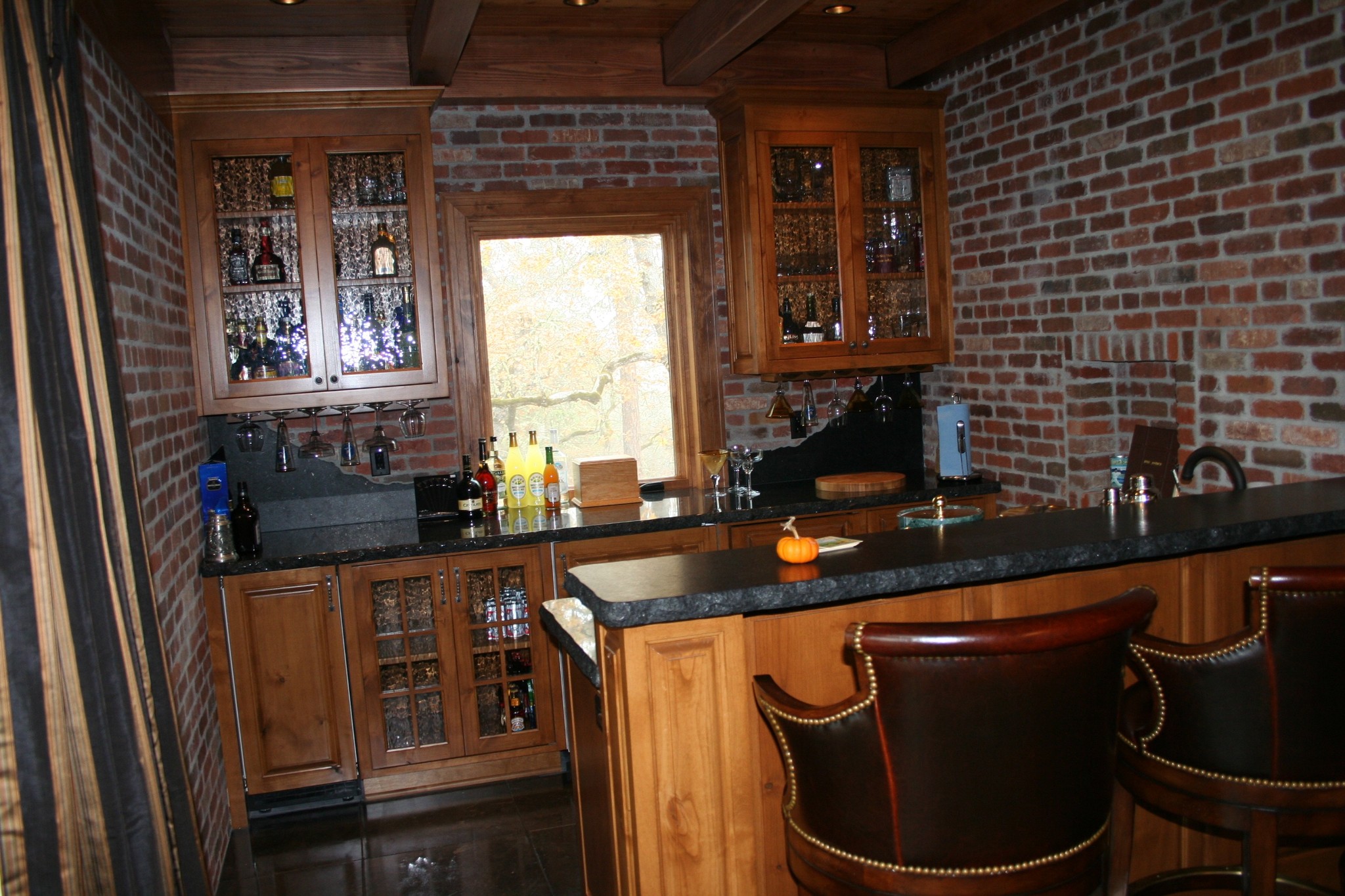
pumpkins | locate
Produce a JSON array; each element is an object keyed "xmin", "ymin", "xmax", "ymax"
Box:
[
  {"xmin": 776, "ymin": 517, "xmax": 819, "ymax": 563},
  {"xmin": 777, "ymin": 562, "xmax": 820, "ymax": 583}
]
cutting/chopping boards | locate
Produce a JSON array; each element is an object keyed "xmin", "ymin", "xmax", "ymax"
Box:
[{"xmin": 814, "ymin": 473, "xmax": 906, "ymax": 491}]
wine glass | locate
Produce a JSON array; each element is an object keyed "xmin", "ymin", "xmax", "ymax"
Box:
[
  {"xmin": 230, "ymin": 413, "xmax": 264, "ymax": 451},
  {"xmin": 331, "ymin": 406, "xmax": 360, "ymax": 466},
  {"xmin": 704, "ymin": 495, "xmax": 753, "ymax": 514},
  {"xmin": 394, "ymin": 400, "xmax": 425, "ymax": 438},
  {"xmin": 298, "ymin": 409, "xmax": 335, "ymax": 458},
  {"xmin": 765, "ymin": 374, "xmax": 915, "ymax": 426},
  {"xmin": 264, "ymin": 411, "xmax": 297, "ymax": 473},
  {"xmin": 359, "ymin": 404, "xmax": 398, "ymax": 453},
  {"xmin": 698, "ymin": 446, "xmax": 762, "ymax": 495}
]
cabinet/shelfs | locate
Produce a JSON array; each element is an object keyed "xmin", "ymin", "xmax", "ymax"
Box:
[
  {"xmin": 203, "ymin": 494, "xmax": 995, "ymax": 831},
  {"xmin": 167, "ymin": 87, "xmax": 450, "ymax": 417},
  {"xmin": 705, "ymin": 84, "xmax": 954, "ymax": 382}
]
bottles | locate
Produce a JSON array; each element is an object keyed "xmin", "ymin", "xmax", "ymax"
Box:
[
  {"xmin": 457, "ymin": 429, "xmax": 568, "ymax": 520},
  {"xmin": 495, "ymin": 681, "xmax": 536, "ymax": 732},
  {"xmin": 229, "ymin": 229, "xmax": 248, "ymax": 286},
  {"xmin": 373, "ymin": 223, "xmax": 397, "ymax": 276},
  {"xmin": 1100, "ymin": 487, "xmax": 1123, "ymax": 506},
  {"xmin": 268, "ymin": 156, "xmax": 294, "ymax": 209},
  {"xmin": 228, "ymin": 317, "xmax": 300, "ymax": 381},
  {"xmin": 253, "ymin": 219, "xmax": 283, "ymax": 284},
  {"xmin": 461, "ymin": 514, "xmax": 571, "ymax": 537},
  {"xmin": 338, "ymin": 286, "xmax": 419, "ymax": 372},
  {"xmin": 230, "ymin": 482, "xmax": 262, "ymax": 557},
  {"xmin": 1126, "ymin": 475, "xmax": 1162, "ymax": 501},
  {"xmin": 779, "ymin": 297, "xmax": 842, "ymax": 345}
]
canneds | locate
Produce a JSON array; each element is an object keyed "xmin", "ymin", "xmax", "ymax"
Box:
[{"xmin": 485, "ymin": 587, "xmax": 529, "ymax": 641}]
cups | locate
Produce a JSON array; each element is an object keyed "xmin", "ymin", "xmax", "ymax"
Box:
[{"xmin": 893, "ymin": 307, "xmax": 927, "ymax": 339}]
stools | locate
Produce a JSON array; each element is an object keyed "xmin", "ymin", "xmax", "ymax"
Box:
[{"xmin": 750, "ymin": 566, "xmax": 1345, "ymax": 896}]
